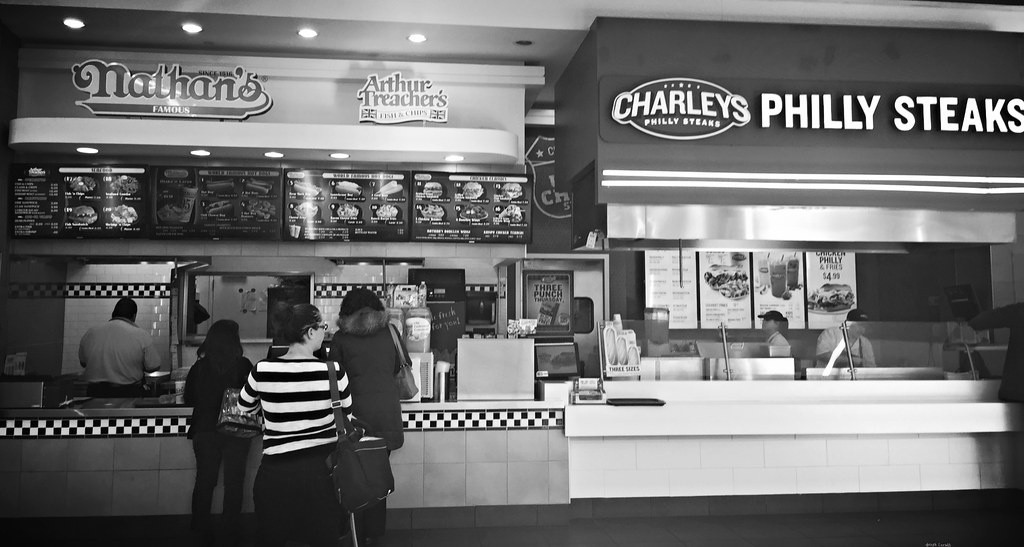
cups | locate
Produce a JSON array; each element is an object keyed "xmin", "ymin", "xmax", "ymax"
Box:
[
  {"xmin": 771, "ymin": 262, "xmax": 787, "ymax": 298},
  {"xmin": 787, "ymin": 258, "xmax": 799, "ymax": 288},
  {"xmin": 175, "ymin": 395, "xmax": 185, "ymax": 404},
  {"xmin": 176, "ymin": 184, "xmax": 198, "ymax": 223},
  {"xmin": 175, "ymin": 381, "xmax": 185, "ymax": 394},
  {"xmin": 731, "ymin": 253, "xmax": 747, "ymax": 269},
  {"xmin": 758, "ymin": 258, "xmax": 771, "ymax": 287}
]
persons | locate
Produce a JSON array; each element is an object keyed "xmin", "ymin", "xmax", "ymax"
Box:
[
  {"xmin": 237, "ymin": 300, "xmax": 354, "ymax": 547},
  {"xmin": 759, "ymin": 311, "xmax": 791, "ymax": 357},
  {"xmin": 815, "ymin": 308, "xmax": 877, "ymax": 368},
  {"xmin": 181, "ymin": 318, "xmax": 261, "ymax": 547},
  {"xmin": 78, "ymin": 297, "xmax": 162, "ymax": 399},
  {"xmin": 969, "ymin": 299, "xmax": 1024, "ymax": 512},
  {"xmin": 328, "ymin": 288, "xmax": 413, "ymax": 547}
]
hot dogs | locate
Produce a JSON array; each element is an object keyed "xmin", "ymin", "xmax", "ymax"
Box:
[
  {"xmin": 293, "ymin": 179, "xmax": 323, "ymax": 197},
  {"xmin": 244, "ymin": 180, "xmax": 273, "ymax": 195},
  {"xmin": 335, "ymin": 180, "xmax": 363, "ymax": 196},
  {"xmin": 204, "ymin": 178, "xmax": 236, "ymax": 194},
  {"xmin": 203, "ymin": 199, "xmax": 233, "ymax": 217}
]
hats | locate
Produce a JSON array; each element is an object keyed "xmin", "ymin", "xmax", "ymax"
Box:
[
  {"xmin": 757, "ymin": 311, "xmax": 783, "ymax": 321},
  {"xmin": 847, "ymin": 309, "xmax": 868, "ymax": 321}
]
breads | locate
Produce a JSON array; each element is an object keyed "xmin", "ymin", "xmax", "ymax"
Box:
[{"xmin": 606, "ymin": 327, "xmax": 639, "ymax": 366}]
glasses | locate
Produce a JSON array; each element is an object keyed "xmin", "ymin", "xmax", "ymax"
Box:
[{"xmin": 306, "ymin": 324, "xmax": 328, "ymax": 330}]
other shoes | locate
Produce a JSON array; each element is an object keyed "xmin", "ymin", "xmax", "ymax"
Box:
[{"xmin": 362, "ymin": 536, "xmax": 377, "ymax": 546}]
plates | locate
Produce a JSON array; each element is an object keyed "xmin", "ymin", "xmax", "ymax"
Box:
[
  {"xmin": 808, "ymin": 309, "xmax": 854, "ymax": 314},
  {"xmin": 460, "ymin": 211, "xmax": 489, "ymax": 221}
]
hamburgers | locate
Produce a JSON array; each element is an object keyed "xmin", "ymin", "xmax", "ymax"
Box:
[
  {"xmin": 67, "ymin": 204, "xmax": 98, "ymax": 226},
  {"xmin": 807, "ymin": 283, "xmax": 855, "ymax": 312},
  {"xmin": 501, "ymin": 183, "xmax": 525, "ymax": 200},
  {"xmin": 703, "ymin": 262, "xmax": 750, "ymax": 300},
  {"xmin": 424, "ymin": 180, "xmax": 444, "ymax": 200},
  {"xmin": 462, "ymin": 182, "xmax": 484, "ymax": 199}
]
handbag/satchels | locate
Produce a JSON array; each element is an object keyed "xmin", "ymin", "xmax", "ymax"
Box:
[
  {"xmin": 215, "ymin": 388, "xmax": 263, "ymax": 439},
  {"xmin": 331, "ymin": 418, "xmax": 396, "ymax": 517},
  {"xmin": 395, "ymin": 363, "xmax": 419, "ymax": 400}
]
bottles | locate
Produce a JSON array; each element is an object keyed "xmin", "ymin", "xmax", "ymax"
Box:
[
  {"xmin": 603, "ymin": 321, "xmax": 617, "ymax": 364},
  {"xmin": 418, "ymin": 281, "xmax": 427, "ymax": 308},
  {"xmin": 628, "ymin": 342, "xmax": 639, "ymax": 366},
  {"xmin": 616, "ymin": 329, "xmax": 627, "ymax": 365}
]
